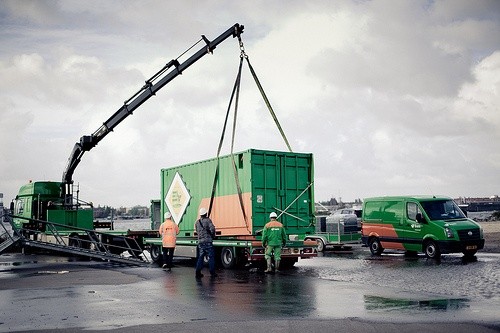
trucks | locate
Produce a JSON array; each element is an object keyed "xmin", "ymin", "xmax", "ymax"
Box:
[{"xmin": 289, "ymin": 232, "xmax": 362, "ymax": 252}]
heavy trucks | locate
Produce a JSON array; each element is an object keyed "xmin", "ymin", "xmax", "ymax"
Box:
[{"xmin": 10, "ymin": 23, "xmax": 244, "ymax": 256}]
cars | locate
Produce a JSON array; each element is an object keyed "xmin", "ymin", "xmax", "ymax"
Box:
[{"xmin": 327, "ymin": 209, "xmax": 361, "ymax": 231}]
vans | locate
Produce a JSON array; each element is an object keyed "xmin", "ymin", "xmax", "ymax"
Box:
[{"xmin": 361, "ymin": 196, "xmax": 485, "ymax": 258}]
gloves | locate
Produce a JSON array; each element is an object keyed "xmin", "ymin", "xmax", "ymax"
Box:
[
  {"xmin": 282, "ymin": 242, "xmax": 286, "ymax": 247},
  {"xmin": 262, "ymin": 244, "xmax": 266, "ymax": 249}
]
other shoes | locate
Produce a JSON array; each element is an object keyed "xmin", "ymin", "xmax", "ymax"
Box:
[
  {"xmin": 168, "ymin": 266, "xmax": 171, "ymax": 271},
  {"xmin": 195, "ymin": 274, "xmax": 204, "ymax": 278},
  {"xmin": 162, "ymin": 264, "xmax": 167, "ymax": 268},
  {"xmin": 211, "ymin": 273, "xmax": 219, "ymax": 277}
]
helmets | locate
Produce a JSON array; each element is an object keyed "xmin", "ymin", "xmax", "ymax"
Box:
[
  {"xmin": 164, "ymin": 212, "xmax": 171, "ymax": 219},
  {"xmin": 199, "ymin": 208, "xmax": 207, "ymax": 215},
  {"xmin": 270, "ymin": 212, "xmax": 277, "ymax": 218}
]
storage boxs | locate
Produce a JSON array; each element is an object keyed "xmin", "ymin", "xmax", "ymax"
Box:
[{"xmin": 160, "ymin": 147, "xmax": 314, "ymax": 235}]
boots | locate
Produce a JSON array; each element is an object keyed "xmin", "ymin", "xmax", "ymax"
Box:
[
  {"xmin": 264, "ymin": 258, "xmax": 272, "ymax": 272},
  {"xmin": 275, "ymin": 260, "xmax": 280, "ymax": 273}
]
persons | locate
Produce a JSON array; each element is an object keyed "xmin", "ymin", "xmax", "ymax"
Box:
[
  {"xmin": 194, "ymin": 208, "xmax": 222, "ymax": 279},
  {"xmin": 158, "ymin": 211, "xmax": 180, "ymax": 272},
  {"xmin": 261, "ymin": 212, "xmax": 287, "ymax": 272}
]
